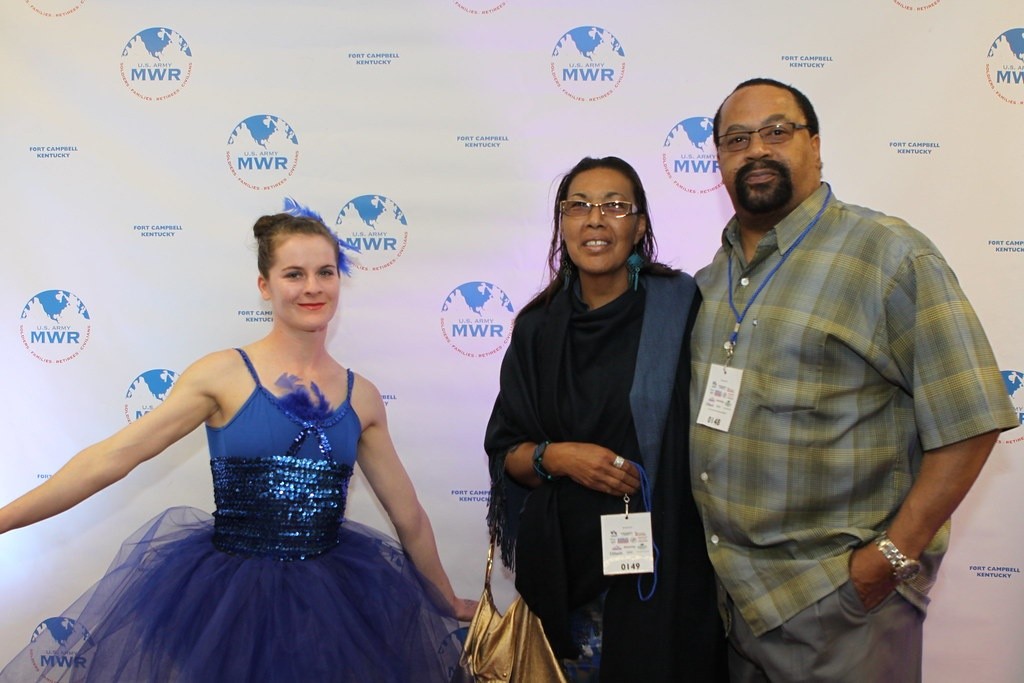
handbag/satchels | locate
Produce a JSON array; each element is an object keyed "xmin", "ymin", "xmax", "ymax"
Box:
[{"xmin": 459, "ymin": 505, "xmax": 566, "ymax": 683}]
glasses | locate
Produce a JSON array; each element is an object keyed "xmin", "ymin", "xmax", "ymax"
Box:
[
  {"xmin": 715, "ymin": 122, "xmax": 810, "ymax": 152},
  {"xmin": 560, "ymin": 199, "xmax": 638, "ymax": 218}
]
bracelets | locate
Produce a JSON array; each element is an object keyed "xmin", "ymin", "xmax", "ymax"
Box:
[{"xmin": 532, "ymin": 441, "xmax": 556, "ymax": 483}]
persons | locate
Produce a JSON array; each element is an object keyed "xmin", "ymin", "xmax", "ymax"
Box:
[
  {"xmin": 1, "ymin": 214, "xmax": 480, "ymax": 683},
  {"xmin": 485, "ymin": 155, "xmax": 729, "ymax": 683},
  {"xmin": 686, "ymin": 77, "xmax": 1020, "ymax": 683}
]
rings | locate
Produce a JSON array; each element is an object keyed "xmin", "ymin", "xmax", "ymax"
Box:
[{"xmin": 613, "ymin": 456, "xmax": 625, "ymax": 468}]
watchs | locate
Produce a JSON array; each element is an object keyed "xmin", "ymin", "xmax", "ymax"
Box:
[{"xmin": 874, "ymin": 531, "xmax": 921, "ymax": 582}]
